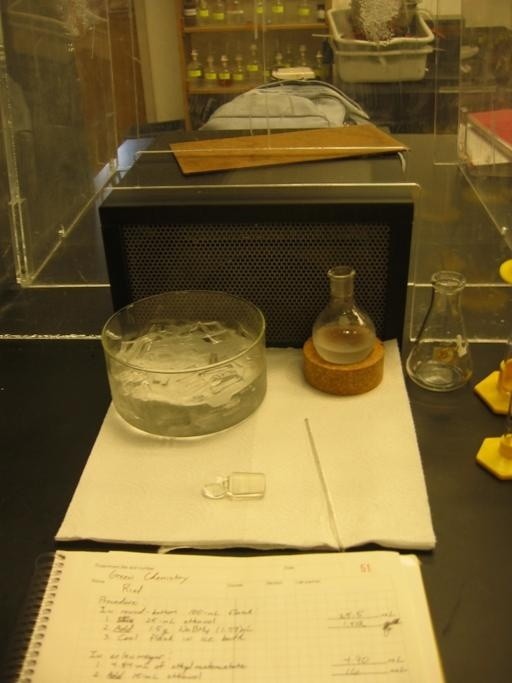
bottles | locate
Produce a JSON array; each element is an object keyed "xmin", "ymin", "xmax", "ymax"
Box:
[
  {"xmin": 406, "ymin": 269, "xmax": 474, "ymax": 395},
  {"xmin": 187, "ymin": 43, "xmax": 331, "ymax": 88},
  {"xmin": 184, "ymin": 0, "xmax": 326, "ymax": 28},
  {"xmin": 311, "ymin": 265, "xmax": 376, "ymax": 364}
]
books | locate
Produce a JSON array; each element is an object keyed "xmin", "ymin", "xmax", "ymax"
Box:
[{"xmin": 1, "ymin": 546, "xmax": 447, "ymax": 682}]
